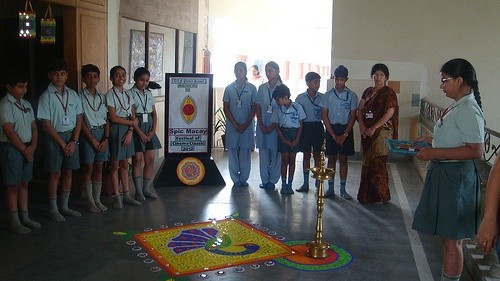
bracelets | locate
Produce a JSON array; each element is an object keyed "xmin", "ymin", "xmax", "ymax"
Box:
[
  {"xmin": 104, "ymin": 137, "xmax": 108, "ymax": 139},
  {"xmin": 128, "ymin": 128, "xmax": 134, "ymax": 132}
]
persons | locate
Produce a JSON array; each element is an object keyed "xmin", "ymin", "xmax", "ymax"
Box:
[
  {"xmin": 295, "ymin": 72, "xmax": 324, "ymax": 192},
  {"xmin": 270, "ymin": 84, "xmax": 306, "ymax": 193},
  {"xmin": 79, "ymin": 64, "xmax": 110, "ymax": 212},
  {"xmin": 222, "ymin": 61, "xmax": 256, "ymax": 187},
  {"xmin": 254, "ymin": 61, "xmax": 283, "ymax": 187},
  {"xmin": 411, "ymin": 59, "xmax": 484, "ymax": 281},
  {"xmin": 247, "ymin": 65, "xmax": 266, "ymax": 92},
  {"xmin": 0, "ymin": 58, "xmax": 82, "ymax": 234},
  {"xmin": 130, "ymin": 68, "xmax": 162, "ymax": 200},
  {"xmin": 319, "ymin": 65, "xmax": 358, "ymax": 199},
  {"xmin": 105, "ymin": 66, "xmax": 140, "ymax": 209},
  {"xmin": 357, "ymin": 63, "xmax": 399, "ymax": 207},
  {"xmin": 477, "ymin": 149, "xmax": 500, "ymax": 263}
]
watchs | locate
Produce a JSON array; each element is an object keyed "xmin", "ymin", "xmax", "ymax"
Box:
[{"xmin": 72, "ymin": 139, "xmax": 79, "ymax": 144}]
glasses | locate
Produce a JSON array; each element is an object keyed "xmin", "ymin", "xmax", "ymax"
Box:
[{"xmin": 441, "ymin": 77, "xmax": 457, "ymax": 84}]
